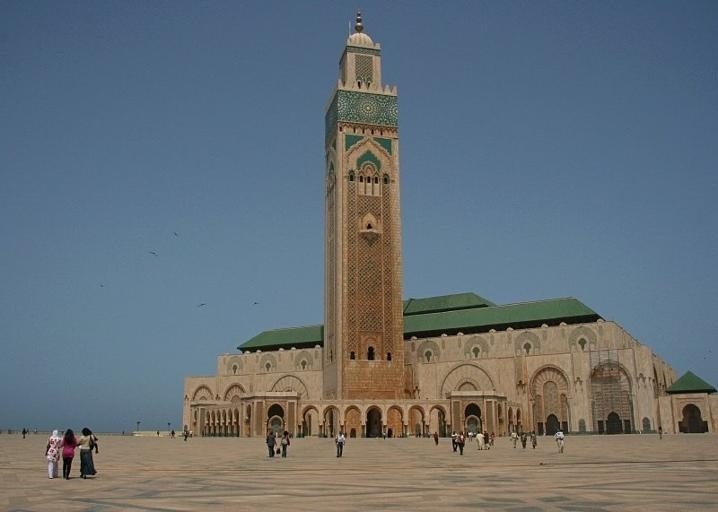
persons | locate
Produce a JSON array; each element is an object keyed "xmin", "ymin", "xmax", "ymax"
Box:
[
  {"xmin": 416, "ymin": 431, "xmax": 438, "ymax": 446},
  {"xmin": 22, "ymin": 427, "xmax": 27, "ymax": 439},
  {"xmin": 171, "ymin": 429, "xmax": 175, "ymax": 439},
  {"xmin": 659, "ymin": 426, "xmax": 663, "ymax": 439},
  {"xmin": 157, "ymin": 430, "xmax": 159, "ymax": 437},
  {"xmin": 264, "ymin": 431, "xmax": 293, "ymax": 458},
  {"xmin": 451, "ymin": 429, "xmax": 538, "ymax": 455},
  {"xmin": 183, "ymin": 424, "xmax": 193, "ymax": 441},
  {"xmin": 335, "ymin": 430, "xmax": 345, "ymax": 458},
  {"xmin": 383, "ymin": 432, "xmax": 385, "ymax": 440},
  {"xmin": 44, "ymin": 427, "xmax": 99, "ymax": 480},
  {"xmin": 553, "ymin": 429, "xmax": 565, "ymax": 453}
]
